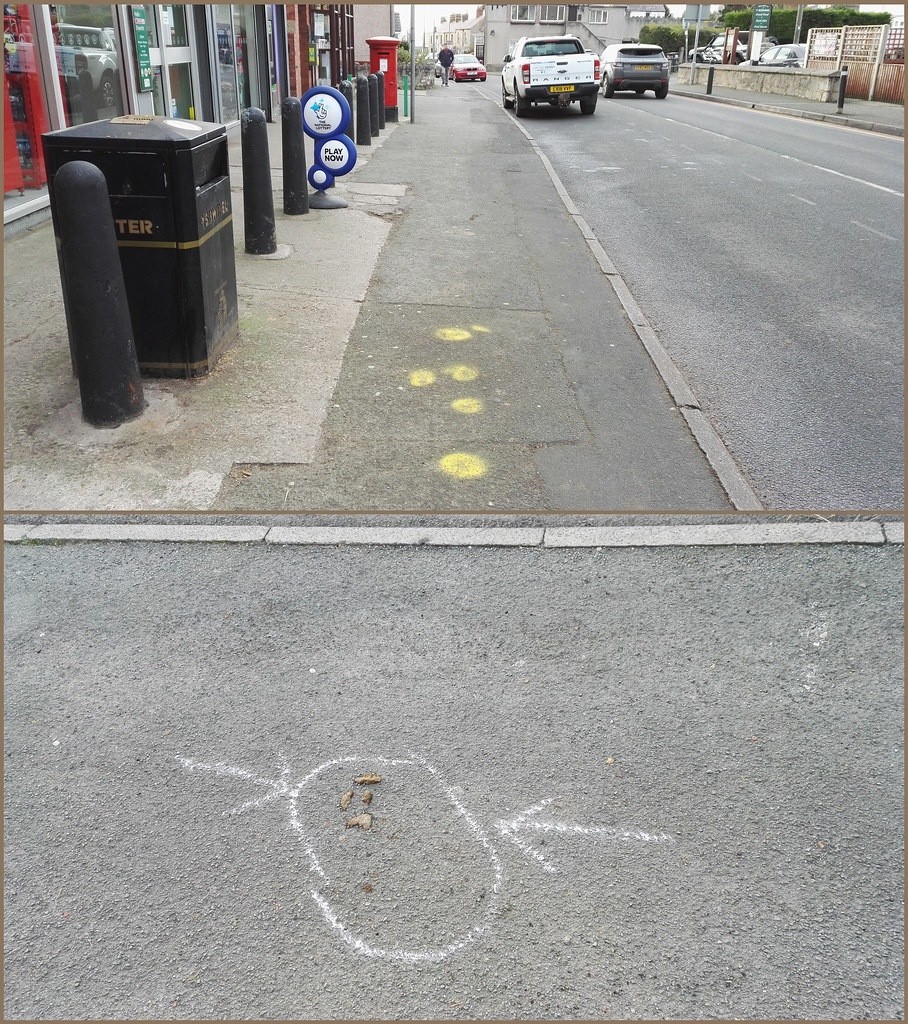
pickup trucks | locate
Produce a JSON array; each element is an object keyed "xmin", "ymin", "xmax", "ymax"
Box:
[{"xmin": 500, "ymin": 34, "xmax": 601, "ymax": 119}]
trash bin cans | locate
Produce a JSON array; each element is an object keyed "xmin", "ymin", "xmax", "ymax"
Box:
[{"xmin": 42, "ymin": 113, "xmax": 239, "ymax": 379}]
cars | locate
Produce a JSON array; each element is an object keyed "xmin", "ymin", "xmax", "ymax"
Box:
[
  {"xmin": 689, "ymin": 31, "xmax": 776, "ymax": 65},
  {"xmin": 448, "ymin": 54, "xmax": 487, "ymax": 82},
  {"xmin": 434, "ymin": 60, "xmax": 443, "ymax": 78},
  {"xmin": 740, "ymin": 43, "xmax": 807, "ymax": 68},
  {"xmin": 600, "ymin": 42, "xmax": 671, "ymax": 100}
]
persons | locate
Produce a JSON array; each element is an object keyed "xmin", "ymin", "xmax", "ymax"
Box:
[{"xmin": 438, "ymin": 44, "xmax": 454, "ymax": 87}]
